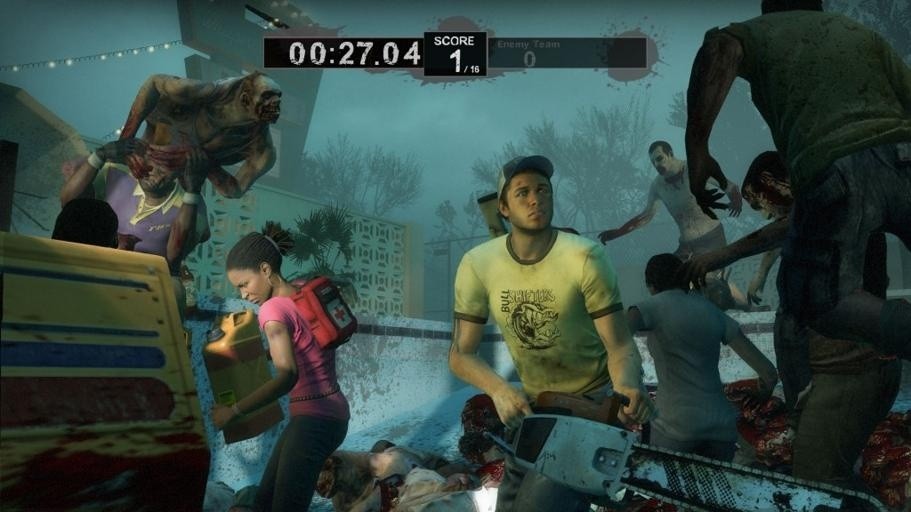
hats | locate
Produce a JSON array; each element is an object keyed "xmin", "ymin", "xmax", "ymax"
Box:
[
  {"xmin": 497, "ymin": 154, "xmax": 554, "ymax": 210},
  {"xmin": 53, "ymin": 197, "xmax": 119, "ymax": 248}
]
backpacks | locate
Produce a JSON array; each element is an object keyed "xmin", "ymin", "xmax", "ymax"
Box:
[{"xmin": 292, "ymin": 275, "xmax": 358, "ymax": 351}]
